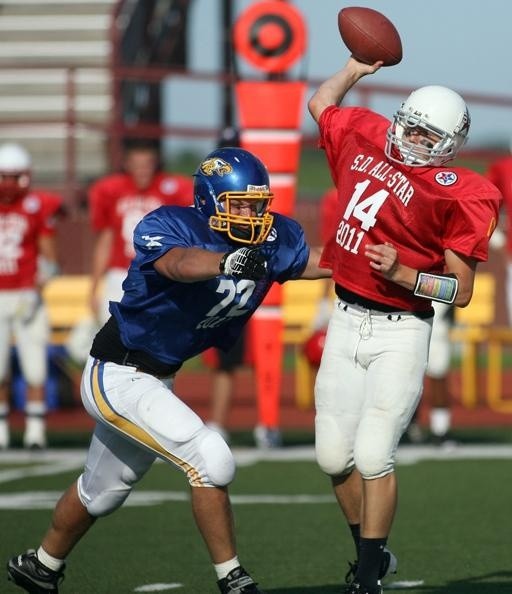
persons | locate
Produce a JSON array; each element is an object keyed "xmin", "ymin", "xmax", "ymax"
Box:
[
  {"xmin": 0, "ymin": 141, "xmax": 67, "ymax": 449},
  {"xmin": 87, "ymin": 137, "xmax": 198, "ymax": 331},
  {"xmin": 481, "ymin": 154, "xmax": 512, "ymax": 327},
  {"xmin": 6, "ymin": 146, "xmax": 333, "ymax": 594},
  {"xmin": 406, "ymin": 294, "xmax": 457, "ymax": 447},
  {"xmin": 307, "ymin": 55, "xmax": 502, "ymax": 594},
  {"xmin": 203, "ymin": 327, "xmax": 253, "ymax": 448}
]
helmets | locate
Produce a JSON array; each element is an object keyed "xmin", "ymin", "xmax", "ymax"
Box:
[
  {"xmin": 394, "ymin": 84, "xmax": 470, "ymax": 168},
  {"xmin": 193, "ymin": 147, "xmax": 274, "ymax": 244}
]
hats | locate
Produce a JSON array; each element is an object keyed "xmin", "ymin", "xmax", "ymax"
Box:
[{"xmin": 0, "ymin": 146, "xmax": 29, "ymax": 177}]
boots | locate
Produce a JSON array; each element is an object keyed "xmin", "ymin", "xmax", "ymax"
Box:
[
  {"xmin": 1, "ymin": 403, "xmax": 10, "ymax": 446},
  {"xmin": 22, "ymin": 403, "xmax": 47, "ymax": 448}
]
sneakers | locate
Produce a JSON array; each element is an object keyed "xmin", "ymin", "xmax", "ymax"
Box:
[
  {"xmin": 5, "ymin": 548, "xmax": 65, "ymax": 594},
  {"xmin": 346, "ymin": 545, "xmax": 398, "ymax": 584},
  {"xmin": 346, "ymin": 583, "xmax": 383, "ymax": 594},
  {"xmin": 216, "ymin": 568, "xmax": 260, "ymax": 593}
]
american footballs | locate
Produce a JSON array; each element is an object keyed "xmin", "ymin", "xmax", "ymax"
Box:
[{"xmin": 338, "ymin": 6, "xmax": 403, "ymax": 67}]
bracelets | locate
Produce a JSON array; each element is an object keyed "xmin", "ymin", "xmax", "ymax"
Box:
[{"xmin": 413, "ymin": 270, "xmax": 459, "ymax": 306}]
